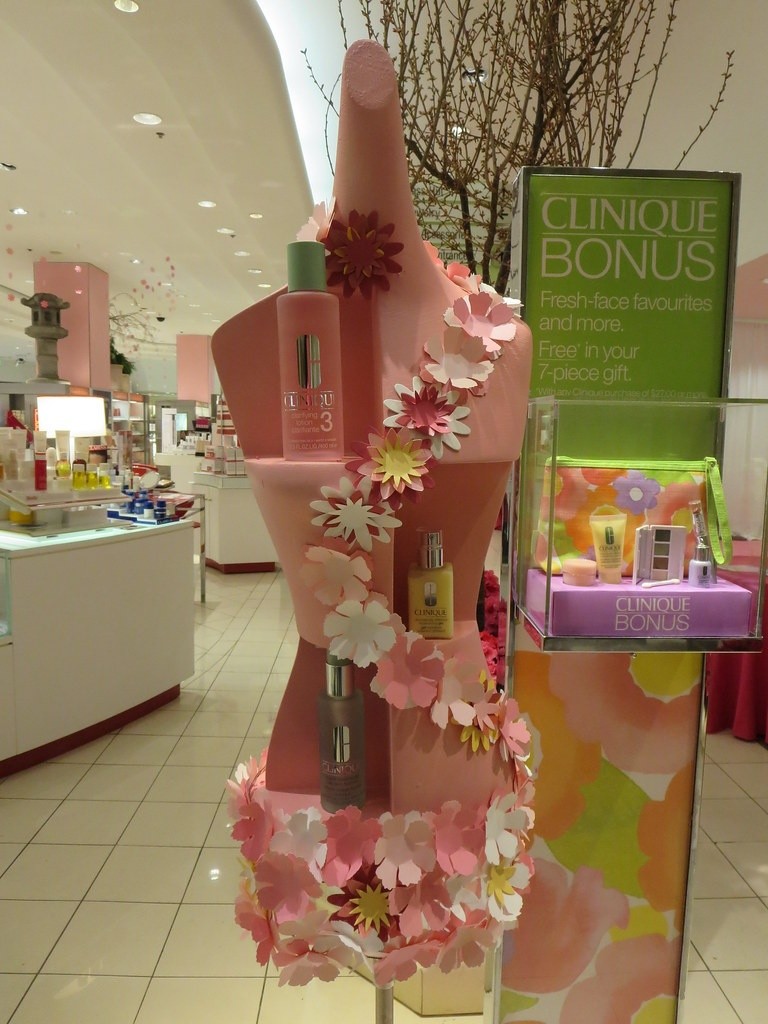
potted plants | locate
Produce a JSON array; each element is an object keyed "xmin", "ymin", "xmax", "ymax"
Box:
[
  {"xmin": 110, "ymin": 336, "xmax": 123, "ymax": 389},
  {"xmin": 121, "ymin": 360, "xmax": 136, "ymax": 392}
]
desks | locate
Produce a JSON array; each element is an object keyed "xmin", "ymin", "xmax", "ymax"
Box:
[{"xmin": 704, "ymin": 540, "xmax": 768, "ymax": 744}]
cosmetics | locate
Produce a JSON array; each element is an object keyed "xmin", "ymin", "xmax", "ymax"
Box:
[
  {"xmin": 319, "ymin": 658, "xmax": 367, "ymax": 812},
  {"xmin": 564, "ymin": 513, "xmax": 719, "ymax": 588},
  {"xmin": 405, "ymin": 531, "xmax": 454, "ymax": 640},
  {"xmin": 278, "ymin": 241, "xmax": 346, "ymax": 461},
  {"xmin": 0, "ymin": 407, "xmax": 247, "ymax": 536}
]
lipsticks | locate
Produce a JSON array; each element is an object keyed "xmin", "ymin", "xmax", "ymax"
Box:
[{"xmin": 685, "ymin": 501, "xmax": 707, "ymax": 537}]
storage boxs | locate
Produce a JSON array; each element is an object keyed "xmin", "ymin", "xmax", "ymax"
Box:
[{"xmin": 200, "ymin": 445, "xmax": 246, "ymax": 475}]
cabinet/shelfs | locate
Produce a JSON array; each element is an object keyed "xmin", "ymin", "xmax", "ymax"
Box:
[
  {"xmin": 514, "ymin": 393, "xmax": 768, "ymax": 653},
  {"xmin": 154, "ymin": 488, "xmax": 206, "ymax": 603},
  {"xmin": 154, "ymin": 416, "xmax": 211, "ymax": 553},
  {"xmin": 189, "ymin": 390, "xmax": 279, "ymax": 575},
  {"xmin": 113, "ymin": 391, "xmax": 151, "ymax": 465},
  {"xmin": 0, "ymin": 383, "xmax": 196, "ymax": 777}
]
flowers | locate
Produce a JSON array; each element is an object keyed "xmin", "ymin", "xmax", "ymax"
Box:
[{"xmin": 224, "ymin": 194, "xmax": 536, "ymax": 986}]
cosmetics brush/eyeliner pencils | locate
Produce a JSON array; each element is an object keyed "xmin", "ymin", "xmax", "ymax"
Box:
[{"xmin": 642, "ymin": 578, "xmax": 679, "ymax": 588}]
